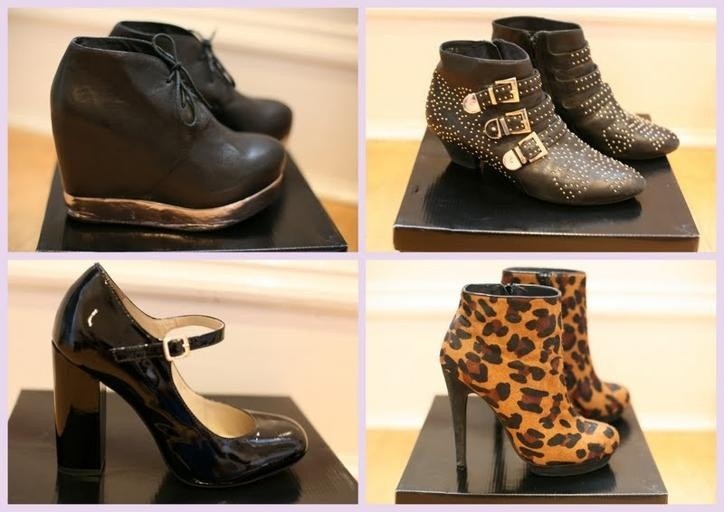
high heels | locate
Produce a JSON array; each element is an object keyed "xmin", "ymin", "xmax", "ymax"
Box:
[
  {"xmin": 437, "ymin": 279, "xmax": 622, "ymax": 478},
  {"xmin": 422, "ymin": 38, "xmax": 651, "ymax": 209},
  {"xmin": 47, "ymin": 34, "xmax": 290, "ymax": 238},
  {"xmin": 489, "ymin": 13, "xmax": 681, "ymax": 162},
  {"xmin": 493, "ymin": 264, "xmax": 631, "ymax": 455},
  {"xmin": 108, "ymin": 15, "xmax": 291, "ymax": 145},
  {"xmin": 46, "ymin": 260, "xmax": 314, "ymax": 491}
]
